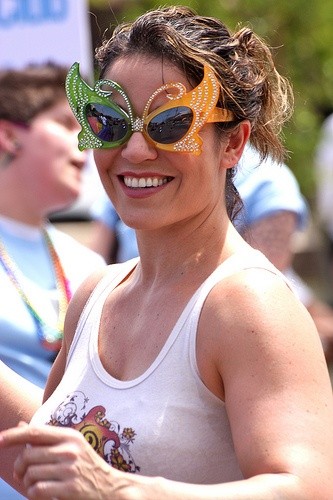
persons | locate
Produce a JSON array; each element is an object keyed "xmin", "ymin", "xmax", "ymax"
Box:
[
  {"xmin": 0, "ymin": 4, "xmax": 333, "ymax": 499},
  {"xmin": 1, "ymin": 57, "xmax": 109, "ymax": 499},
  {"xmin": 275, "ymin": 265, "xmax": 332, "ymax": 370},
  {"xmin": 88, "ymin": 131, "xmax": 310, "ymax": 296}
]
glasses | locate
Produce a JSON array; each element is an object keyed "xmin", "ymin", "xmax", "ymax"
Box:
[{"xmin": 65, "ymin": 62, "xmax": 237, "ymax": 155}]
